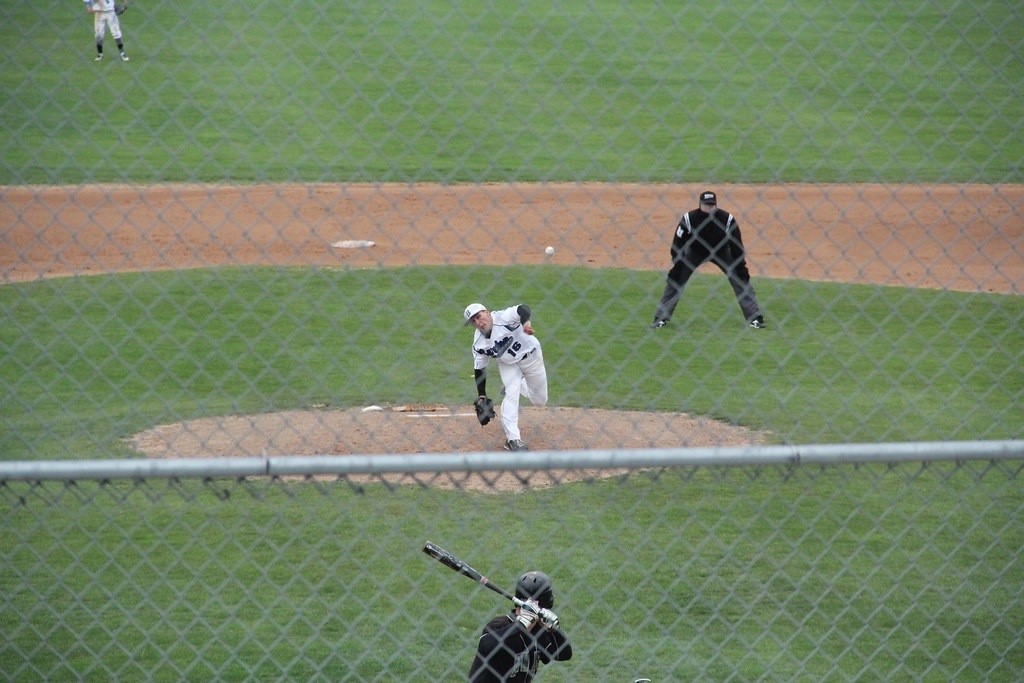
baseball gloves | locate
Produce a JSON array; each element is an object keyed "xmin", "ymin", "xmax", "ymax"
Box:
[
  {"xmin": 474, "ymin": 397, "xmax": 496, "ymax": 425},
  {"xmin": 115, "ymin": 3, "xmax": 127, "ymax": 16}
]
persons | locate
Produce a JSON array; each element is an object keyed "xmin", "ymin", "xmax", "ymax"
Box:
[
  {"xmin": 84, "ymin": 0, "xmax": 129, "ymax": 61},
  {"xmin": 652, "ymin": 191, "xmax": 766, "ymax": 329},
  {"xmin": 468, "ymin": 571, "xmax": 572, "ymax": 683},
  {"xmin": 464, "ymin": 304, "xmax": 548, "ymax": 452}
]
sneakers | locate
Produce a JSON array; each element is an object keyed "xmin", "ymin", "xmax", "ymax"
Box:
[
  {"xmin": 650, "ymin": 320, "xmax": 668, "ymax": 328},
  {"xmin": 121, "ymin": 51, "xmax": 129, "ymax": 61},
  {"xmin": 95, "ymin": 54, "xmax": 104, "ymax": 61},
  {"xmin": 503, "ymin": 440, "xmax": 529, "ymax": 453},
  {"xmin": 750, "ymin": 316, "xmax": 766, "ymax": 328}
]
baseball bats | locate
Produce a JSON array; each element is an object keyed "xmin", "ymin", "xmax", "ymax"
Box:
[{"xmin": 421, "ymin": 541, "xmax": 560, "ymax": 631}]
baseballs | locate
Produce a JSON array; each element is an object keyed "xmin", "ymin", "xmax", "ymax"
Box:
[{"xmin": 545, "ymin": 247, "xmax": 554, "ymax": 256}]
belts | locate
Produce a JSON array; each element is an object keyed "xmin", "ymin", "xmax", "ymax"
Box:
[{"xmin": 520, "ymin": 348, "xmax": 536, "ymax": 360}]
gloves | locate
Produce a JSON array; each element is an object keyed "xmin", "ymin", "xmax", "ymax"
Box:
[
  {"xmin": 669, "ymin": 266, "xmax": 680, "ymax": 279},
  {"xmin": 739, "ymin": 266, "xmax": 750, "ymax": 281},
  {"xmin": 517, "ymin": 599, "xmax": 541, "ymax": 632},
  {"xmin": 539, "ymin": 608, "xmax": 561, "ymax": 633}
]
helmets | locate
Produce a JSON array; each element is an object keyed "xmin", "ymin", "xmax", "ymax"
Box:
[{"xmin": 516, "ymin": 571, "xmax": 554, "ymax": 610}]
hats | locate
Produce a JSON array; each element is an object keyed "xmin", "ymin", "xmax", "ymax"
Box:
[
  {"xmin": 463, "ymin": 303, "xmax": 487, "ymax": 327},
  {"xmin": 699, "ymin": 191, "xmax": 717, "ymax": 204}
]
